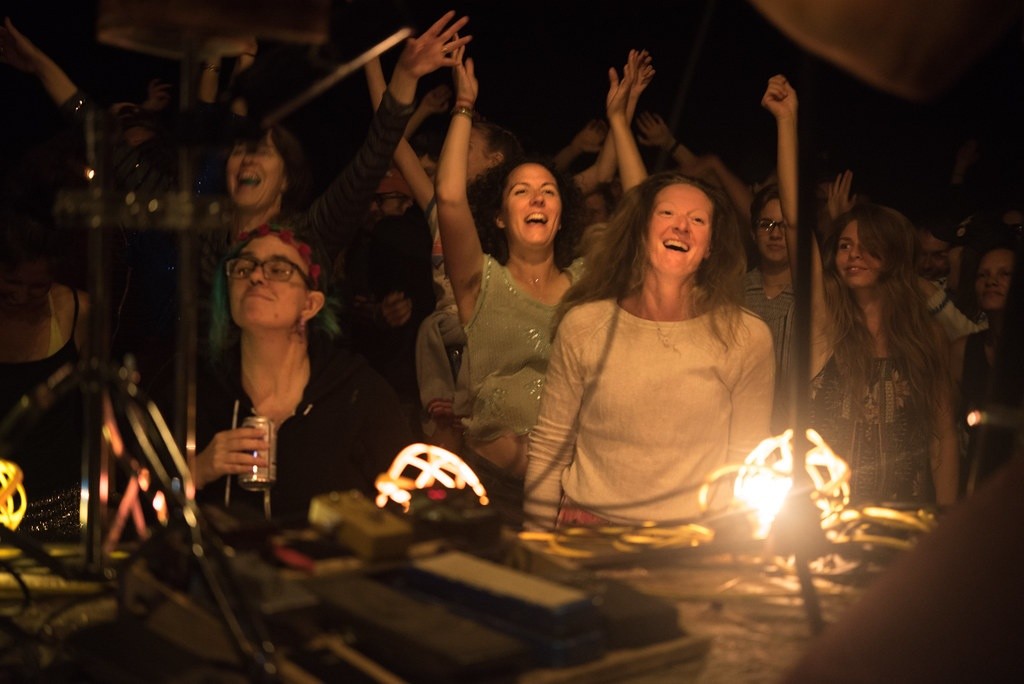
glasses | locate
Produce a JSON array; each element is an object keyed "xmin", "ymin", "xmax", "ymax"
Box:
[
  {"xmin": 224, "ymin": 254, "xmax": 315, "ymax": 290},
  {"xmin": 755, "ymin": 218, "xmax": 787, "ymax": 233}
]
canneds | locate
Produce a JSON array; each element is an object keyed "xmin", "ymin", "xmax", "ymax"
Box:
[{"xmin": 238, "ymin": 416, "xmax": 278, "ymax": 490}]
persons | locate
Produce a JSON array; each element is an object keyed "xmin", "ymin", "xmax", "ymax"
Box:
[{"xmin": 1, "ymin": 9, "xmax": 1024, "ymax": 552}]
